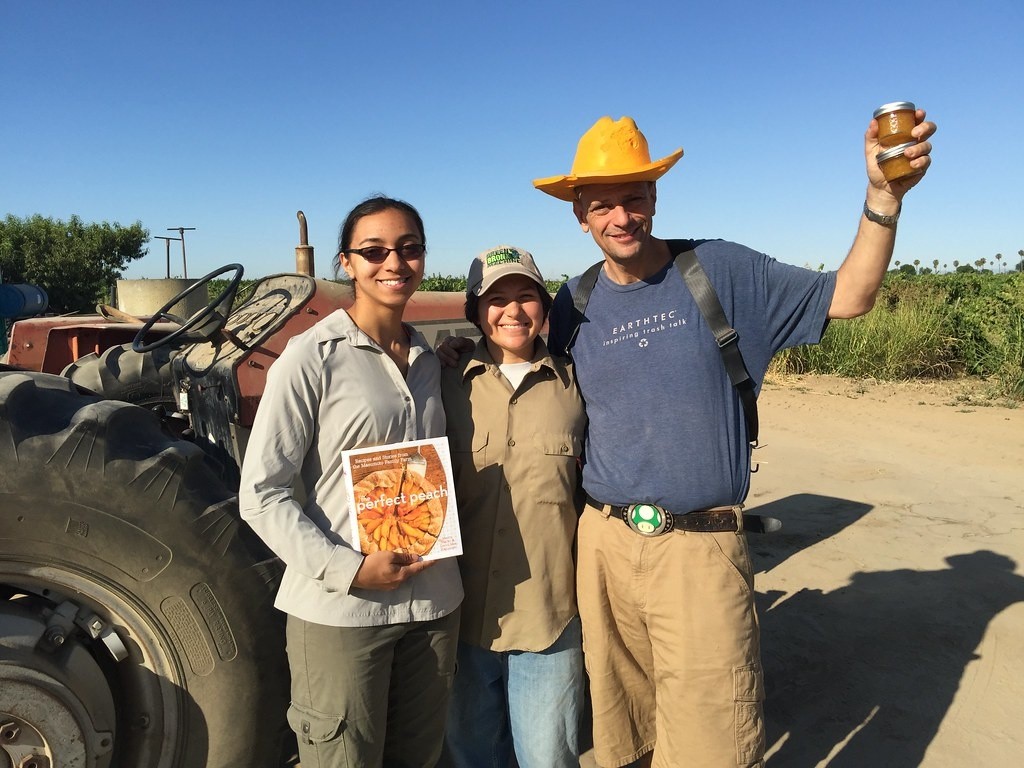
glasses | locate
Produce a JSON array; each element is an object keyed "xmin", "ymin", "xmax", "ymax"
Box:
[{"xmin": 342, "ymin": 244, "xmax": 425, "ymax": 264}]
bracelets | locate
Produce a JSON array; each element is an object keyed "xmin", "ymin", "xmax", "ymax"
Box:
[{"xmin": 864, "ymin": 200, "xmax": 902, "ymax": 225}]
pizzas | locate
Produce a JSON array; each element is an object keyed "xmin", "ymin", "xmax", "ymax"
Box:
[{"xmin": 354, "ymin": 469, "xmax": 443, "ymax": 558}]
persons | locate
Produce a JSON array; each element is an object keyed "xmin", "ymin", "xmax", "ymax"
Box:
[
  {"xmin": 239, "ymin": 198, "xmax": 467, "ymax": 768},
  {"xmin": 435, "ymin": 109, "xmax": 936, "ymax": 768}
]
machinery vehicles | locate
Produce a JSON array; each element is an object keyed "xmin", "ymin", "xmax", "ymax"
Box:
[{"xmin": 0, "ymin": 211, "xmax": 559, "ymax": 768}]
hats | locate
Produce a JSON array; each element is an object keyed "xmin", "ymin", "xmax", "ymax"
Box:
[
  {"xmin": 533, "ymin": 116, "xmax": 684, "ymax": 201},
  {"xmin": 466, "ymin": 245, "xmax": 548, "ymax": 301}
]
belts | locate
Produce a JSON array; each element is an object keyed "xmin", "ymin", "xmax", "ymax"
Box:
[{"xmin": 583, "ymin": 492, "xmax": 782, "ymax": 537}]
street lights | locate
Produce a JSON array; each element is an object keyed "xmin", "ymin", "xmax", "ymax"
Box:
[
  {"xmin": 166, "ymin": 227, "xmax": 196, "ymax": 279},
  {"xmin": 154, "ymin": 236, "xmax": 182, "ymax": 279}
]
